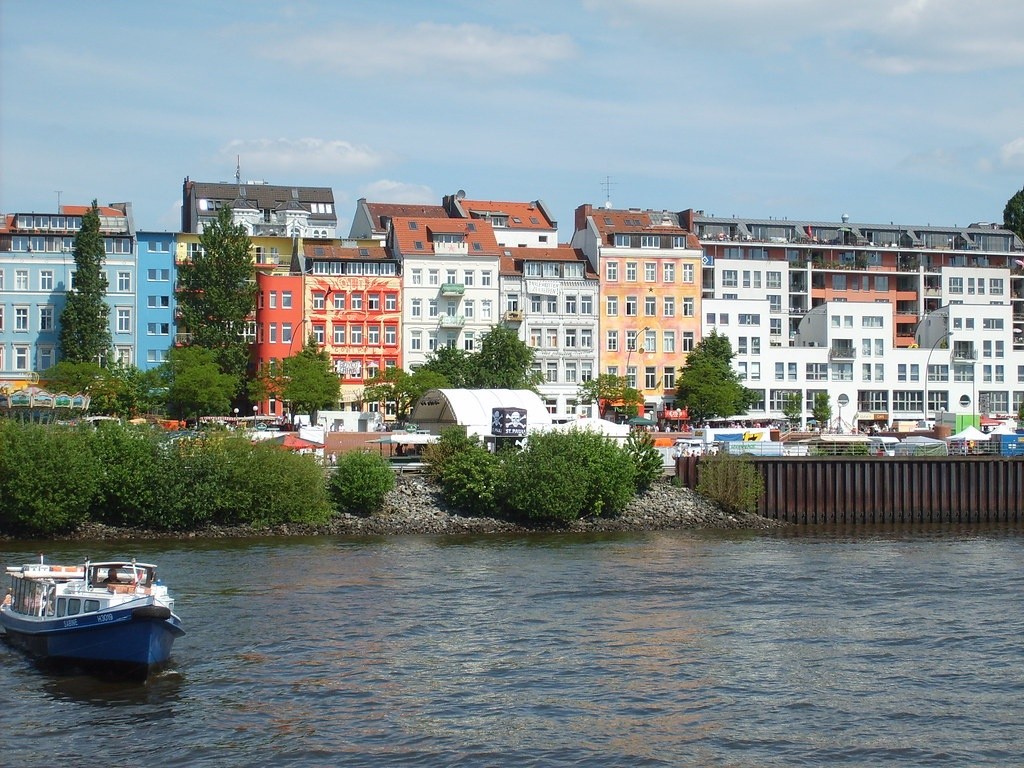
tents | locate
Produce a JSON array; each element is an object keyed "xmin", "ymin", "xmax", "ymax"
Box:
[
  {"xmin": 946, "ymin": 425, "xmax": 990, "ymax": 456},
  {"xmin": 275, "ymin": 434, "xmax": 327, "ymax": 449},
  {"xmin": 896, "ymin": 435, "xmax": 949, "ymax": 457},
  {"xmin": 629, "ymin": 416, "xmax": 656, "ymax": 432},
  {"xmin": 987, "ymin": 424, "xmax": 1017, "ymax": 438}
]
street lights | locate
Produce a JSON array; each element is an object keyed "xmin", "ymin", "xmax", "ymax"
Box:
[
  {"xmin": 234, "ymin": 408, "xmax": 240, "ymax": 426},
  {"xmin": 626, "ymin": 326, "xmax": 651, "ymax": 382},
  {"xmin": 924, "ymin": 332, "xmax": 954, "ymax": 422},
  {"xmin": 253, "ymin": 405, "xmax": 258, "ymax": 428},
  {"xmin": 288, "ymin": 318, "xmax": 308, "ymax": 357}
]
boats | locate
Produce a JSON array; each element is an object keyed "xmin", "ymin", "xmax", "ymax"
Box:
[{"xmin": 0, "ymin": 553, "xmax": 188, "ymax": 687}]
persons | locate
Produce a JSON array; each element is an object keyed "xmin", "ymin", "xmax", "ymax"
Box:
[
  {"xmin": 928, "ymin": 423, "xmax": 935, "ymax": 430},
  {"xmin": 665, "ymin": 425, "xmax": 670, "ymax": 433},
  {"xmin": 375, "ymin": 422, "xmax": 392, "ymax": 432},
  {"xmin": 103, "ymin": 569, "xmax": 121, "ymax": 583},
  {"xmin": 330, "ymin": 451, "xmax": 337, "ymax": 466},
  {"xmin": 280, "ymin": 411, "xmax": 288, "ymax": 431},
  {"xmin": 731, "ymin": 420, "xmax": 856, "ymax": 435},
  {"xmin": 861, "ymin": 422, "xmax": 893, "ymax": 436},
  {"xmin": 26, "ymin": 588, "xmax": 52, "ymax": 611},
  {"xmin": 395, "ymin": 443, "xmax": 403, "ymax": 456},
  {"xmin": 681, "ymin": 421, "xmax": 693, "ymax": 432}
]
